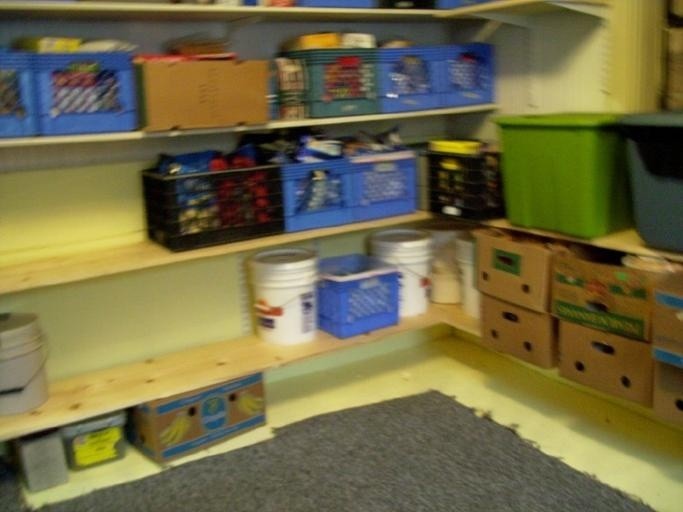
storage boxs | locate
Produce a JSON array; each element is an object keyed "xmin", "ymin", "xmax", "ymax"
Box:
[
  {"xmin": 124, "ymin": 374, "xmax": 265, "ymax": 465},
  {"xmin": 134, "ymin": 59, "xmax": 268, "ymax": 130},
  {"xmin": 62, "ymin": 411, "xmax": 126, "ymax": 471},
  {"xmin": 476, "ymin": 232, "xmax": 550, "ymax": 313},
  {"xmin": 478, "ymin": 294, "xmax": 559, "ymax": 369},
  {"xmin": 622, "ymin": 113, "xmax": 683, "ymax": 249},
  {"xmin": 547, "ymin": 248, "xmax": 651, "ymax": 343},
  {"xmin": 553, "ymin": 316, "xmax": 653, "ymax": 407},
  {"xmin": 649, "ymin": 263, "xmax": 681, "ymax": 346},
  {"xmin": 16, "ymin": 433, "xmax": 68, "ymax": 493},
  {"xmin": 490, "ymin": 115, "xmax": 632, "ymax": 239},
  {"xmin": 652, "ymin": 349, "xmax": 681, "ymax": 426}
]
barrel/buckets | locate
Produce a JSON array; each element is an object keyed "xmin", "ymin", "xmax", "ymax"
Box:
[
  {"xmin": 1, "ymin": 314, "xmax": 50, "ymax": 416},
  {"xmin": 369, "ymin": 227, "xmax": 433, "ymax": 320},
  {"xmin": 248, "ymin": 248, "xmax": 321, "ymax": 346}
]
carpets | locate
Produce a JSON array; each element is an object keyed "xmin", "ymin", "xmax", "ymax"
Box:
[{"xmin": 32, "ymin": 390, "xmax": 655, "ymax": 511}]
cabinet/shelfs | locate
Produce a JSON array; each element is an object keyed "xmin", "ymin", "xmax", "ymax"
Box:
[{"xmin": 0, "ymin": 0, "xmax": 683, "ymax": 443}]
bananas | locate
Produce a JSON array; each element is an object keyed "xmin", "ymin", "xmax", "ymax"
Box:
[
  {"xmin": 160, "ymin": 411, "xmax": 191, "ymax": 448},
  {"xmin": 238, "ymin": 390, "xmax": 264, "ymax": 416}
]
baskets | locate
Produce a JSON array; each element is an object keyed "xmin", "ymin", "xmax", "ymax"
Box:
[
  {"xmin": 35, "ymin": 54, "xmax": 139, "ymax": 135},
  {"xmin": 315, "ymin": 252, "xmax": 400, "ymax": 342},
  {"xmin": 376, "ymin": 46, "xmax": 441, "ymax": 115},
  {"xmin": 139, "ymin": 157, "xmax": 284, "ymax": 253},
  {"xmin": 2, "ymin": 52, "xmax": 36, "ymax": 140},
  {"xmin": 349, "ymin": 155, "xmax": 416, "ymax": 222},
  {"xmin": 274, "ymin": 48, "xmax": 375, "ymax": 118},
  {"xmin": 440, "ymin": 42, "xmax": 491, "ymax": 106},
  {"xmin": 426, "ymin": 150, "xmax": 504, "ymax": 221},
  {"xmin": 276, "ymin": 159, "xmax": 351, "ymax": 235}
]
